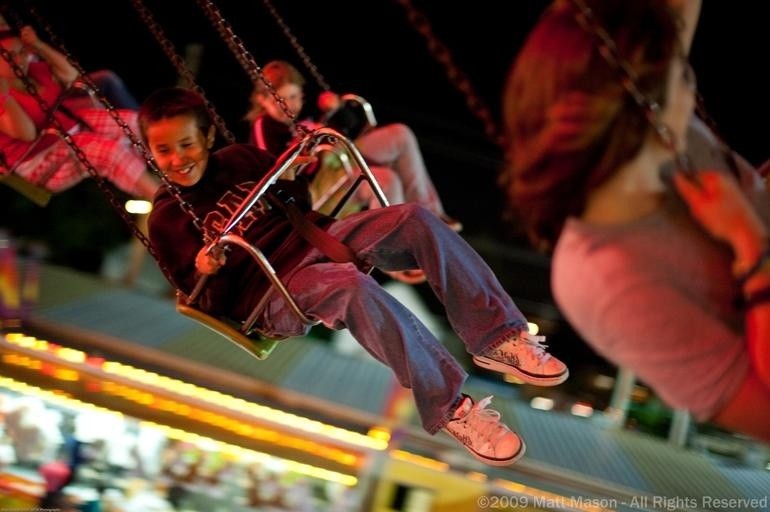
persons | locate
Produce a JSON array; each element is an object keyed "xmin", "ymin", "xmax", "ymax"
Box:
[
  {"xmin": 134, "ymin": 87, "xmax": 569, "ymax": 468},
  {"xmin": 0, "ymin": 26, "xmax": 162, "ymax": 203},
  {"xmin": 38, "ymin": 420, "xmax": 79, "ymax": 511},
  {"xmin": 496, "ymin": 0, "xmax": 770, "ymax": 444},
  {"xmin": 245, "ymin": 60, "xmax": 464, "ymax": 233}
]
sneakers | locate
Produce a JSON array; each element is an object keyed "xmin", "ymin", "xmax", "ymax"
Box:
[
  {"xmin": 385, "ymin": 264, "xmax": 426, "ymax": 285},
  {"xmin": 472, "ymin": 327, "xmax": 570, "ymax": 388},
  {"xmin": 440, "ymin": 393, "xmax": 526, "ymax": 467}
]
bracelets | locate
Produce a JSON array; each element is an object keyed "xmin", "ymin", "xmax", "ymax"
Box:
[
  {"xmin": 740, "ymin": 287, "xmax": 769, "ymax": 310},
  {"xmin": 734, "ymin": 247, "xmax": 770, "ymax": 288}
]
